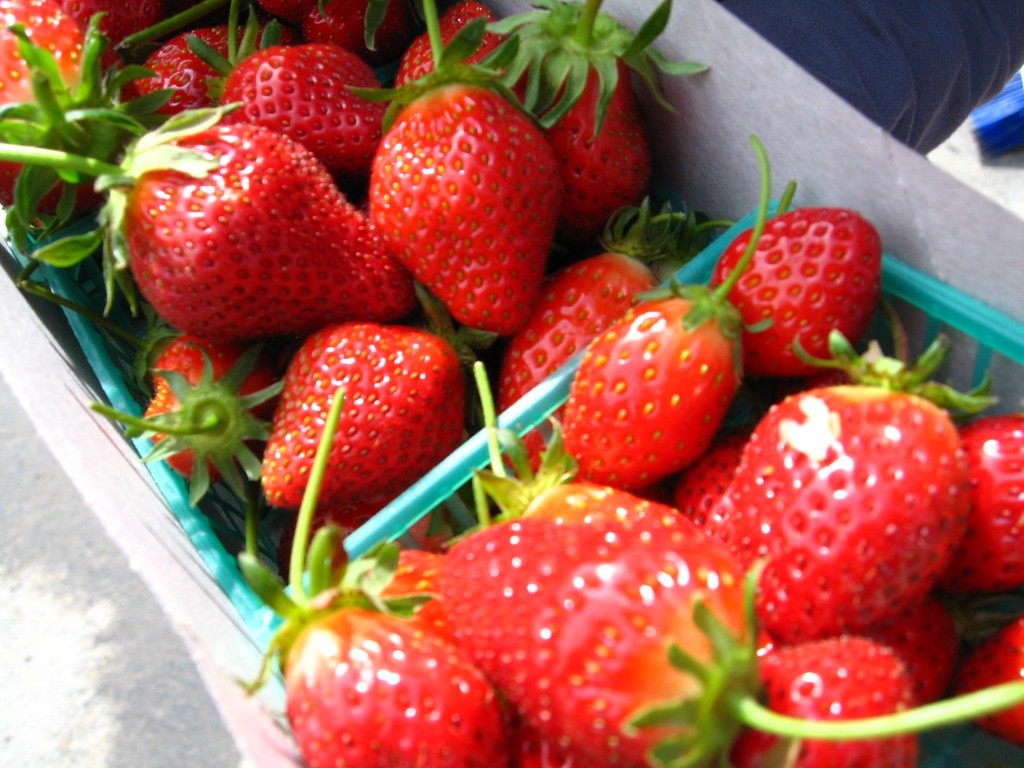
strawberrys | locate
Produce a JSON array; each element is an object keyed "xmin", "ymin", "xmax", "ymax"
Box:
[{"xmin": 0, "ymin": 0, "xmax": 1024, "ymax": 768}]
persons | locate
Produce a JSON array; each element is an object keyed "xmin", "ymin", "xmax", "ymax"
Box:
[{"xmin": 715, "ymin": 0, "xmax": 1024, "ymax": 155}]
